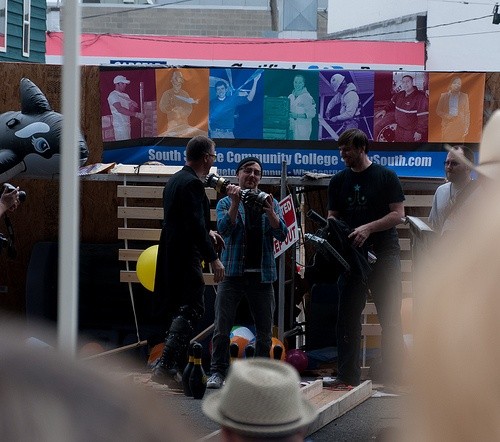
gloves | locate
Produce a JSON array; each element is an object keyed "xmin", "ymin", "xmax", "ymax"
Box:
[{"xmin": 135, "ymin": 112, "xmax": 146, "ymax": 120}]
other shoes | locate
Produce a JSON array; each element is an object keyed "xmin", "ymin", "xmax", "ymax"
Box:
[
  {"xmin": 383, "ymin": 385, "xmax": 398, "ymax": 394},
  {"xmin": 336, "ymin": 378, "xmax": 344, "ymax": 386}
]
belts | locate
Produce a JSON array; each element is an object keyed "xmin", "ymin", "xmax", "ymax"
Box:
[{"xmin": 211, "ymin": 128, "xmax": 233, "ymax": 132}]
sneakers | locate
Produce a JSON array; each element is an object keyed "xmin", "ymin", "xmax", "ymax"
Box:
[
  {"xmin": 207, "ymin": 373, "xmax": 224, "ymax": 388},
  {"xmin": 151, "ymin": 362, "xmax": 183, "ymax": 389}
]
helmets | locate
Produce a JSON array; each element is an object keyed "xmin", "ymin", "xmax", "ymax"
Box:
[{"xmin": 331, "ymin": 74, "xmax": 345, "ymax": 92}]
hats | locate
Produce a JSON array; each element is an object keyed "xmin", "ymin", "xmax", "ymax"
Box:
[
  {"xmin": 236, "ymin": 157, "xmax": 263, "ymax": 180},
  {"xmin": 113, "ymin": 75, "xmax": 131, "ymax": 85},
  {"xmin": 201, "ymin": 358, "xmax": 318, "ymax": 433}
]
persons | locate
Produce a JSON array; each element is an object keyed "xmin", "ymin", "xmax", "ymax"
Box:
[
  {"xmin": 72, "ymin": 107, "xmax": 500, "ymax": 442},
  {"xmin": 209, "ymin": 73, "xmax": 262, "ymax": 138},
  {"xmin": 279, "ymin": 74, "xmax": 316, "ymax": 140},
  {"xmin": 436, "ymin": 76, "xmax": 471, "ymax": 142},
  {"xmin": 376, "ymin": 75, "xmax": 428, "ymax": 142},
  {"xmin": 108, "ymin": 75, "xmax": 146, "ymax": 140},
  {"xmin": 325, "ymin": 73, "xmax": 360, "ymax": 133}
]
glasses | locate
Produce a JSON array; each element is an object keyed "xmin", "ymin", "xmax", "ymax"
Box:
[
  {"xmin": 444, "ymin": 161, "xmax": 459, "ymax": 166},
  {"xmin": 209, "ymin": 154, "xmax": 217, "ymax": 160}
]
